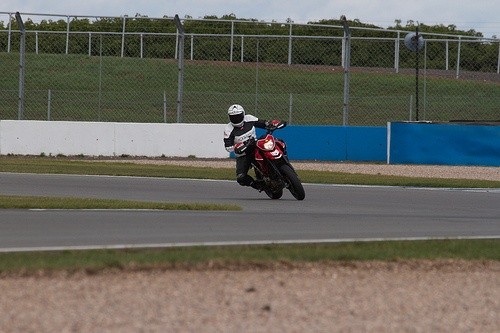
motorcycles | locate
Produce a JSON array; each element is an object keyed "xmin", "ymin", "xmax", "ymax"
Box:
[{"xmin": 235, "ymin": 120, "xmax": 306, "ymax": 202}]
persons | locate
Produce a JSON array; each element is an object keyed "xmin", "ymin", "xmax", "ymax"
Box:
[{"xmin": 223, "ymin": 103, "xmax": 286, "ymax": 193}]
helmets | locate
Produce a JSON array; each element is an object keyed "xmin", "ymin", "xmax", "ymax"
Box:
[{"xmin": 228, "ymin": 104, "xmax": 245, "ymax": 128}]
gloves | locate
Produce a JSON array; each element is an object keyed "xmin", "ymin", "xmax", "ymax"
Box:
[
  {"xmin": 276, "ymin": 120, "xmax": 287, "ymax": 129},
  {"xmin": 235, "ymin": 143, "xmax": 247, "ymax": 154}
]
